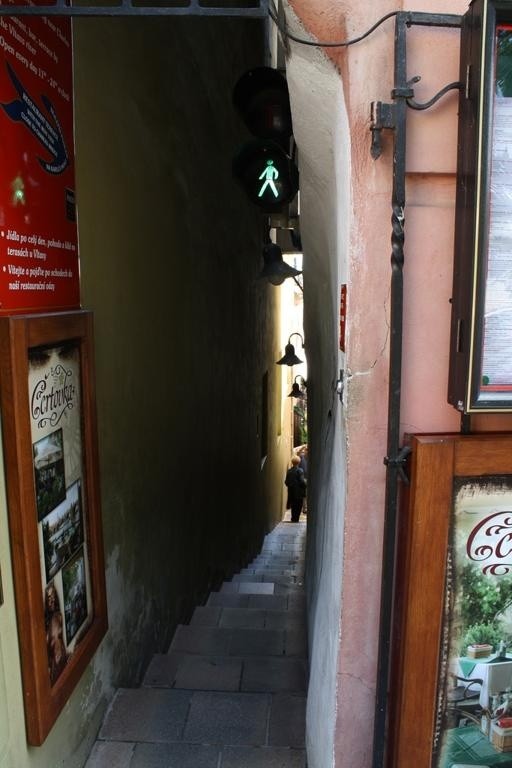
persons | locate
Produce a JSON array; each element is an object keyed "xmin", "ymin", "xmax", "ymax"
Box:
[
  {"xmin": 284, "ymin": 455, "xmax": 306, "ymax": 523},
  {"xmin": 299, "ymin": 448, "xmax": 307, "ymax": 514}
]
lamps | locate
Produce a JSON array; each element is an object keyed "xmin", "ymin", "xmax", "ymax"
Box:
[
  {"xmin": 256, "ymin": 241, "xmax": 304, "ymax": 303},
  {"xmin": 278, "ymin": 331, "xmax": 309, "ymax": 402}
]
